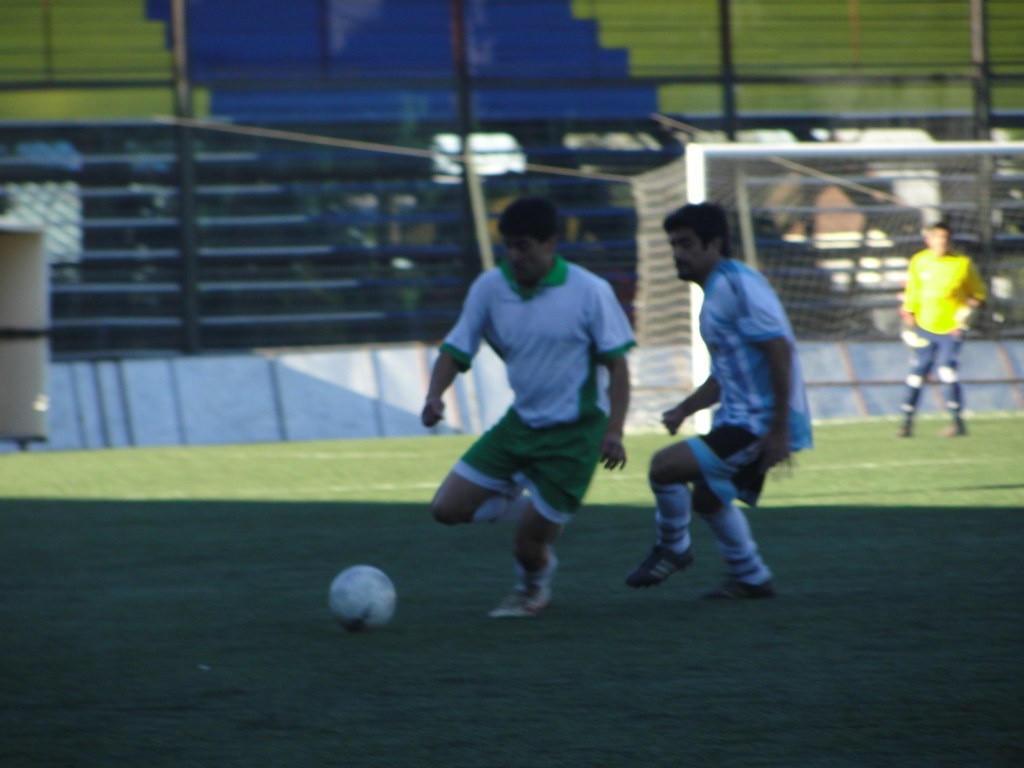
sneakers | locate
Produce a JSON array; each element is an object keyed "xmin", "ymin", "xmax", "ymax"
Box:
[
  {"xmin": 487, "ymin": 581, "xmax": 551, "ymax": 618},
  {"xmin": 624, "ymin": 543, "xmax": 695, "ymax": 588},
  {"xmin": 698, "ymin": 582, "xmax": 776, "ymax": 601},
  {"xmin": 485, "ymin": 489, "xmax": 514, "ymax": 525}
]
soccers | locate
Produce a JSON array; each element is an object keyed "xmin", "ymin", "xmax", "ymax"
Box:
[{"xmin": 327, "ymin": 565, "xmax": 398, "ymax": 631}]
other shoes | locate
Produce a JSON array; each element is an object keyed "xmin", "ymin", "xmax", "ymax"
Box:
[
  {"xmin": 955, "ymin": 419, "xmax": 964, "ymax": 436},
  {"xmin": 902, "ymin": 420, "xmax": 910, "ymax": 436}
]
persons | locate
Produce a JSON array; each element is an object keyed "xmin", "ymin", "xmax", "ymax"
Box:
[
  {"xmin": 898, "ymin": 222, "xmax": 988, "ymax": 437},
  {"xmin": 422, "ymin": 195, "xmax": 638, "ymax": 613},
  {"xmin": 621, "ymin": 203, "xmax": 814, "ymax": 598}
]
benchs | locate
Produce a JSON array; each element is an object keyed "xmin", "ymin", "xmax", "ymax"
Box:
[{"xmin": 0, "ymin": 0, "xmax": 862, "ymax": 348}]
